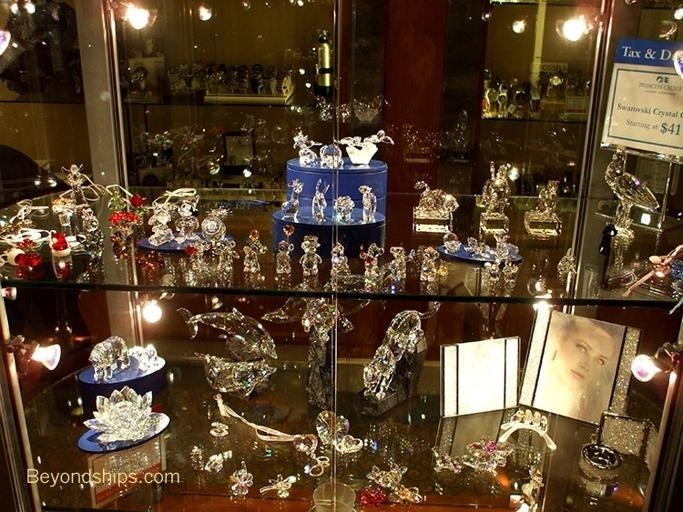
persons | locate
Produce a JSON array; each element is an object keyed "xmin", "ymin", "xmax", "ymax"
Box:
[{"xmin": 548, "ymin": 315, "xmax": 621, "ymax": 420}]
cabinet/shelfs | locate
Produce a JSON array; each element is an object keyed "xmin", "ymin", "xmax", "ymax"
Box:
[{"xmin": 1, "ymin": 184, "xmax": 682, "ymax": 510}]
